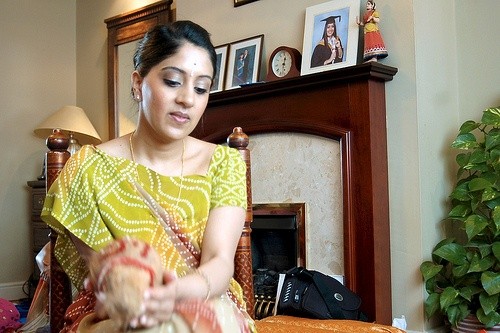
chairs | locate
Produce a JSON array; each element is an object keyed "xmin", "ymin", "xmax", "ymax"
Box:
[{"xmin": 46, "ymin": 124, "xmax": 258, "ymax": 333}]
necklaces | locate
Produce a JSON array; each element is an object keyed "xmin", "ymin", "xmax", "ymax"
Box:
[{"xmin": 130, "ymin": 128, "xmax": 186, "ymax": 212}]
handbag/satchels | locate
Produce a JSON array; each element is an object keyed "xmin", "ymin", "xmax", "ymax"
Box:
[{"xmin": 277, "ymin": 269, "xmax": 362, "ymax": 321}]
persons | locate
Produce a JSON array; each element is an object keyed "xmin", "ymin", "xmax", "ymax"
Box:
[
  {"xmin": 236, "ymin": 49, "xmax": 249, "ymax": 85},
  {"xmin": 311, "ymin": 15, "xmax": 344, "ymax": 68},
  {"xmin": 356, "ymin": 0, "xmax": 389, "ymax": 65},
  {"xmin": 40, "ymin": 20, "xmax": 256, "ymax": 333}
]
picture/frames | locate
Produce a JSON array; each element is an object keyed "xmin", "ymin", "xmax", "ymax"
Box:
[
  {"xmin": 225, "ymin": 33, "xmax": 264, "ymax": 90},
  {"xmin": 208, "ymin": 41, "xmax": 230, "ymax": 93},
  {"xmin": 299, "ymin": 0, "xmax": 361, "ymax": 78}
]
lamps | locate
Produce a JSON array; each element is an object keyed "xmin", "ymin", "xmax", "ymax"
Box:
[{"xmin": 32, "ymin": 104, "xmax": 103, "ymax": 158}]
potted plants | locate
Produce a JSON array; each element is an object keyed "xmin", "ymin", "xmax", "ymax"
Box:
[{"xmin": 421, "ymin": 105, "xmax": 500, "ymax": 333}]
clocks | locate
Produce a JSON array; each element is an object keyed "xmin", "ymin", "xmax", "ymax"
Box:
[{"xmin": 266, "ymin": 44, "xmax": 302, "ymax": 81}]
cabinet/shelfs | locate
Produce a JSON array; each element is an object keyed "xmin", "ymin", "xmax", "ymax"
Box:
[{"xmin": 26, "ymin": 187, "xmax": 51, "ymax": 305}]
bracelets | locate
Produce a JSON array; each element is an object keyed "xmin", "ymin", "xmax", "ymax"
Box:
[
  {"xmin": 191, "ymin": 272, "xmax": 211, "ymax": 303},
  {"xmin": 358, "ymin": 21, "xmax": 361, "ymax": 25}
]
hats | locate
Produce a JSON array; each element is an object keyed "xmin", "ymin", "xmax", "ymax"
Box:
[{"xmin": 320, "ymin": 15, "xmax": 341, "ymax": 24}]
